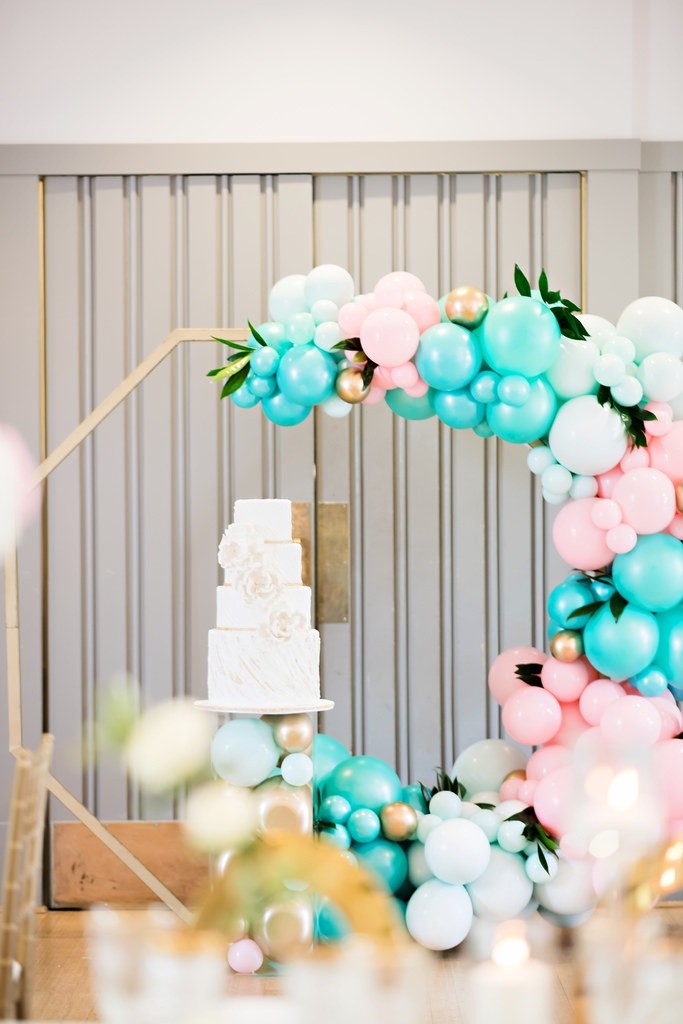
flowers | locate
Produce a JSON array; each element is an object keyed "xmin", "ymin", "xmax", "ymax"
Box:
[{"xmin": 217, "ymin": 521, "xmax": 305, "ymax": 643}]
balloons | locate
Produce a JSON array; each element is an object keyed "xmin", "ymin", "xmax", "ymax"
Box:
[{"xmin": 130, "ymin": 263, "xmax": 683, "ymax": 973}]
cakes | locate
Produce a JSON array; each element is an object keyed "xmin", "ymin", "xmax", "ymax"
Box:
[{"xmin": 207, "ymin": 498, "xmax": 321, "ymax": 706}]
